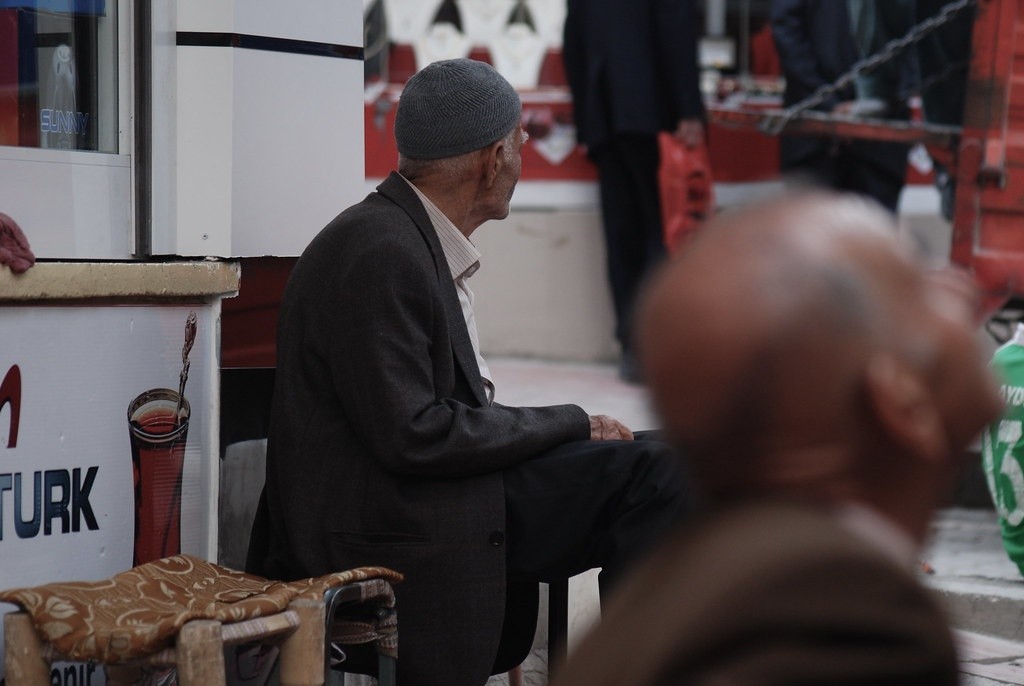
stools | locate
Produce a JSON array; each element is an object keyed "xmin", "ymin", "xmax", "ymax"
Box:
[{"xmin": 0, "ymin": 555, "xmax": 403, "ymax": 686}]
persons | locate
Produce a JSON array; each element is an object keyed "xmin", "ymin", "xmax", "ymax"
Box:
[
  {"xmin": 238, "ymin": 56, "xmax": 685, "ymax": 686},
  {"xmin": 559, "ymin": 189, "xmax": 1005, "ymax": 686},
  {"xmin": 768, "ymin": 0, "xmax": 923, "ymax": 207},
  {"xmin": 559, "ymin": 0, "xmax": 709, "ymax": 385}
]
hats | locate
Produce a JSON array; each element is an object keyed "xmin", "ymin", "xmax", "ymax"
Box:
[{"xmin": 394, "ymin": 57, "xmax": 522, "ymax": 162}]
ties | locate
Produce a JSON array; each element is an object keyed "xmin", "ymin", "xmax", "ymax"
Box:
[{"xmin": 857, "ymin": 0, "xmax": 868, "ymax": 51}]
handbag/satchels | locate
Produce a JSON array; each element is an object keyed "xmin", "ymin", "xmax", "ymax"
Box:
[{"xmin": 659, "ymin": 131, "xmax": 715, "ymax": 258}]
